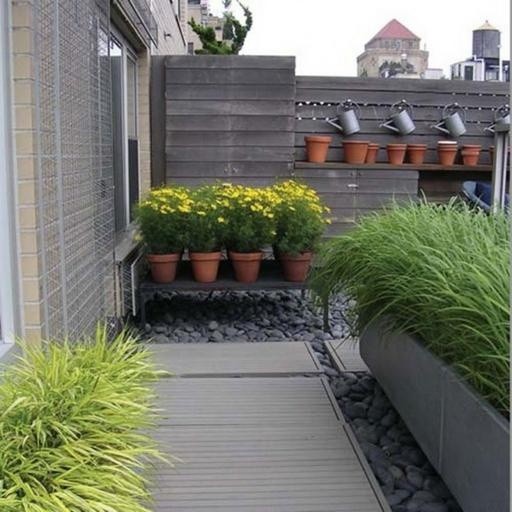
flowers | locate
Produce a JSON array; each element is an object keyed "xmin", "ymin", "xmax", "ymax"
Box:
[{"xmin": 133, "ymin": 178, "xmax": 333, "ymax": 257}]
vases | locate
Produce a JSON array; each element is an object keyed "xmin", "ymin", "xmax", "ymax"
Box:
[
  {"xmin": 304, "ymin": 135, "xmax": 332, "ymax": 162},
  {"xmin": 437, "ymin": 141, "xmax": 482, "ymax": 165},
  {"xmin": 387, "ymin": 144, "xmax": 426, "ymax": 164},
  {"xmin": 341, "ymin": 140, "xmax": 379, "ymax": 164},
  {"xmin": 148, "ymin": 252, "xmax": 313, "ymax": 283}
]
